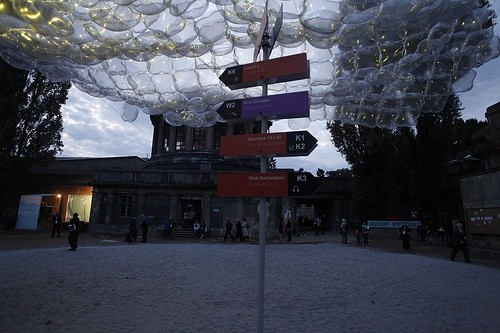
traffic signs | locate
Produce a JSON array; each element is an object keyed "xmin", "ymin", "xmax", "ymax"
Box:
[
  {"xmin": 219, "ymin": 130, "xmax": 319, "ymax": 156},
  {"xmin": 216, "ymin": 90, "xmax": 310, "ymax": 120},
  {"xmin": 216, "ymin": 172, "xmax": 322, "ymax": 195},
  {"xmin": 253, "ymin": 0, "xmax": 269, "ymax": 62},
  {"xmin": 218, "ymin": 52, "xmax": 307, "ymax": 86}
]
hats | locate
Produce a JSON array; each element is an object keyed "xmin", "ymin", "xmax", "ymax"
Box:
[{"xmin": 194, "ymin": 220, "xmax": 199, "ymax": 222}]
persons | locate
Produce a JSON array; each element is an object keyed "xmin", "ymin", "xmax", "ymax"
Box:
[
  {"xmin": 339, "ymin": 219, "xmax": 349, "ymax": 244},
  {"xmin": 352, "ymin": 228, "xmax": 362, "ymax": 247},
  {"xmin": 192, "ymin": 220, "xmax": 208, "ymax": 240},
  {"xmin": 417, "ymin": 223, "xmax": 432, "ymax": 243},
  {"xmin": 284, "ymin": 220, "xmax": 291, "ymax": 241},
  {"xmin": 313, "ymin": 214, "xmax": 327, "ymax": 237},
  {"xmin": 221, "ymin": 216, "xmax": 236, "ymax": 242},
  {"xmin": 68, "ymin": 213, "xmax": 80, "ymax": 251},
  {"xmin": 241, "ymin": 218, "xmax": 249, "ymax": 240},
  {"xmin": 167, "ymin": 216, "xmax": 177, "ymax": 237},
  {"xmin": 139, "ymin": 215, "xmax": 149, "ymax": 243},
  {"xmin": 398, "ymin": 222, "xmax": 411, "ymax": 250},
  {"xmin": 409, "ymin": 208, "xmax": 418, "ymax": 221},
  {"xmin": 232, "ymin": 218, "xmax": 243, "ymax": 242},
  {"xmin": 278, "ymin": 220, "xmax": 283, "ymax": 241},
  {"xmin": 359, "ymin": 221, "xmax": 370, "ymax": 247},
  {"xmin": 50, "ymin": 213, "xmax": 61, "ymax": 239},
  {"xmin": 181, "ymin": 209, "xmax": 202, "ymax": 228},
  {"xmin": 450, "ymin": 223, "xmax": 473, "ymax": 263},
  {"xmin": 129, "ymin": 215, "xmax": 138, "ymax": 242}
]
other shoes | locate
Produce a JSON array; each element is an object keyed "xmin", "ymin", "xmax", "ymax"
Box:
[{"xmin": 68, "ymin": 246, "xmax": 78, "ymax": 251}]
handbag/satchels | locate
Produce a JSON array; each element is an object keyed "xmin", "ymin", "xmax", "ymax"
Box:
[
  {"xmin": 246, "ymin": 224, "xmax": 250, "ymax": 229},
  {"xmin": 340, "ymin": 228, "xmax": 346, "ymax": 234},
  {"xmin": 68, "ymin": 224, "xmax": 76, "ymax": 232}
]
